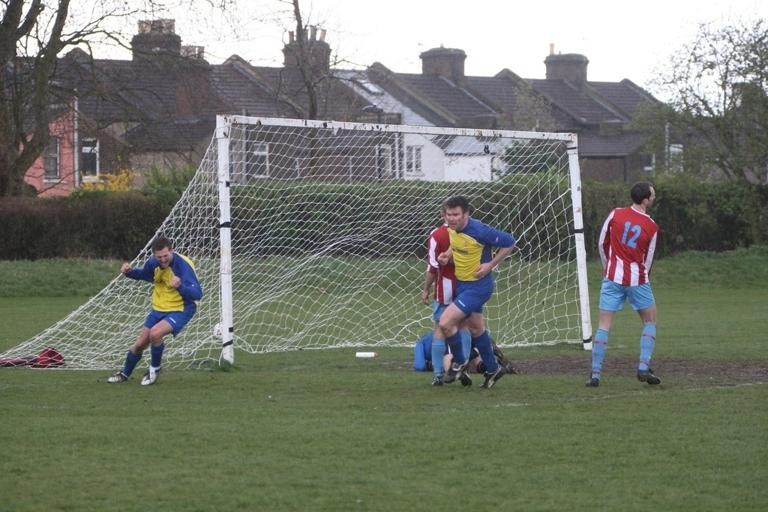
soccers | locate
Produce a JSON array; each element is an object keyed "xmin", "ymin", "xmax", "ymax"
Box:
[{"xmin": 214, "ymin": 322, "xmax": 222, "ymax": 339}]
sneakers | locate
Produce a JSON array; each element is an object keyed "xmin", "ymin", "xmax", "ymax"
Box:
[
  {"xmin": 585, "ymin": 378, "xmax": 600, "ymax": 386},
  {"xmin": 107, "ymin": 370, "xmax": 128, "ymax": 383},
  {"xmin": 432, "ymin": 357, "xmax": 514, "ymax": 389},
  {"xmin": 140, "ymin": 365, "xmax": 161, "ymax": 385},
  {"xmin": 638, "ymin": 369, "xmax": 661, "ymax": 384}
]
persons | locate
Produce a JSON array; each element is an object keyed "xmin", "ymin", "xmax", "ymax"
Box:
[
  {"xmin": 583, "ymin": 181, "xmax": 663, "ymax": 387},
  {"xmin": 107, "ymin": 237, "xmax": 203, "ymax": 386},
  {"xmin": 438, "ymin": 196, "xmax": 516, "ymax": 391},
  {"xmin": 421, "ymin": 200, "xmax": 479, "ymax": 387},
  {"xmin": 412, "ymin": 326, "xmax": 522, "ymax": 376}
]
